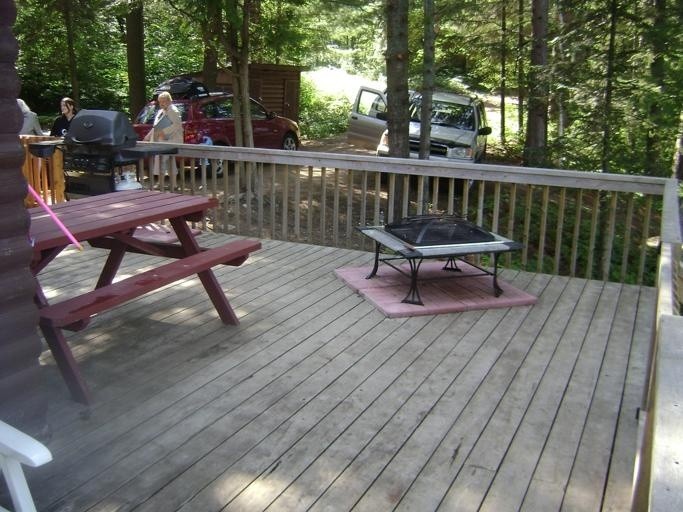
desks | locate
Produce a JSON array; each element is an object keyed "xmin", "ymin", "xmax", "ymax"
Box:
[
  {"xmin": 27, "ymin": 188, "xmax": 241, "ymax": 405},
  {"xmin": 354, "ymin": 220, "xmax": 526, "ymax": 305}
]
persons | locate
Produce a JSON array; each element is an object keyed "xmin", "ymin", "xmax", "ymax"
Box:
[
  {"xmin": 15, "ymin": 98, "xmax": 46, "ymax": 210},
  {"xmin": 49, "ymin": 96, "xmax": 79, "ymax": 140},
  {"xmin": 143, "ymin": 91, "xmax": 185, "ymax": 191}
]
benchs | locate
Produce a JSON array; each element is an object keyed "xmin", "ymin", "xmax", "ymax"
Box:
[{"xmin": 38, "ymin": 239, "xmax": 262, "ymax": 405}]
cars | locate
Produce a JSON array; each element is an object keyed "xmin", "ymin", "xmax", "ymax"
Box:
[
  {"xmin": 132, "ymin": 75, "xmax": 301, "ymax": 178},
  {"xmin": 346, "ymin": 85, "xmax": 492, "ymax": 194}
]
